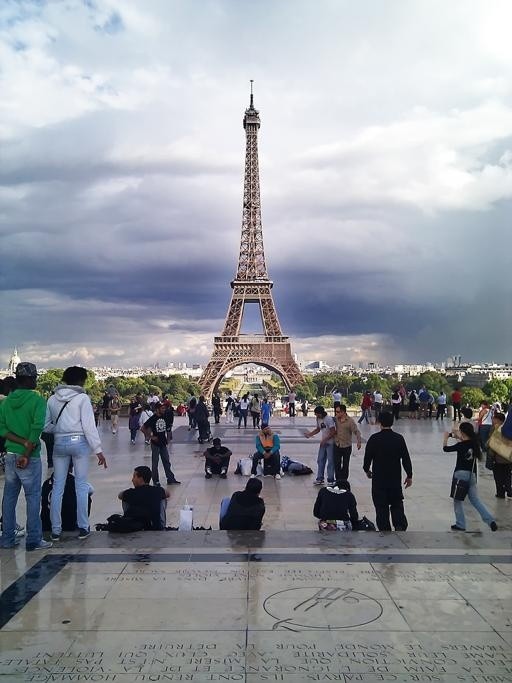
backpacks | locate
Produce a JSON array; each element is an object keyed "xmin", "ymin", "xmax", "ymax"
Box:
[{"xmin": 96, "ymin": 513, "xmax": 145, "ymax": 532}]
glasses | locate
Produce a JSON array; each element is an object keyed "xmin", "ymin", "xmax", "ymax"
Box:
[{"xmin": 335, "ymin": 410, "xmax": 340, "ymax": 413}]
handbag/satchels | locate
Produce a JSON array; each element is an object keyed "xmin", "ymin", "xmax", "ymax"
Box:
[
  {"xmin": 40, "ymin": 471, "xmax": 92, "ymax": 531},
  {"xmin": 358, "ymin": 516, "xmax": 375, "ymax": 531},
  {"xmin": 486, "ymin": 423, "xmax": 511, "ymax": 462},
  {"xmin": 485, "ymin": 455, "xmax": 495, "ymax": 469},
  {"xmin": 450, "ymin": 478, "xmax": 468, "ymax": 500}
]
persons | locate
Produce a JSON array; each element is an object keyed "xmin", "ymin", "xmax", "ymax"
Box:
[
  {"xmin": 363, "ymin": 410, "xmax": 412, "ymax": 531},
  {"xmin": 225, "ymin": 391, "xmax": 272, "ymax": 429},
  {"xmin": 1, "ymin": 361, "xmax": 54, "ymax": 550},
  {"xmin": 141, "ymin": 401, "xmax": 181, "ymax": 486},
  {"xmin": 2, "ymin": 376, "xmax": 15, "ymax": 471},
  {"xmin": 462, "ymin": 401, "xmax": 511, "ymax": 501},
  {"xmin": 205, "ymin": 438, "xmax": 232, "ymax": 479},
  {"xmin": 129, "ymin": 391, "xmax": 224, "ymax": 447},
  {"xmin": 43, "ymin": 365, "xmax": 108, "ymax": 539},
  {"xmin": 285, "ymin": 390, "xmax": 309, "ymax": 417},
  {"xmin": 118, "ymin": 465, "xmax": 170, "ymax": 530},
  {"xmin": 41, "ymin": 458, "xmax": 93, "ymax": 533},
  {"xmin": 442, "ymin": 421, "xmax": 498, "ymax": 531},
  {"xmin": 250, "ymin": 423, "xmax": 282, "ymax": 480},
  {"xmin": 333, "ymin": 389, "xmax": 341, "ymax": 401},
  {"xmin": 1, "ymin": 514, "xmax": 26, "ymax": 537},
  {"xmin": 312, "ymin": 480, "xmax": 359, "ymax": 530},
  {"xmin": 358, "ymin": 383, "xmax": 461, "ymax": 424},
  {"xmin": 220, "ymin": 476, "xmax": 266, "ymax": 529},
  {"xmin": 305, "ymin": 400, "xmax": 362, "ymax": 485},
  {"xmin": 103, "ymin": 384, "xmax": 121, "ymax": 434}
]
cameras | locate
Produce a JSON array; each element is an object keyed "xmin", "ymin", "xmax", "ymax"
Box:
[{"xmin": 448, "ymin": 433, "xmax": 453, "ymax": 437}]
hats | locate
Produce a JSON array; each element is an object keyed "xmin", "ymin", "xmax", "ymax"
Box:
[
  {"xmin": 213, "ymin": 438, "xmax": 221, "ymax": 445},
  {"xmin": 261, "ymin": 423, "xmax": 268, "ymax": 430},
  {"xmin": 154, "ymin": 401, "xmax": 164, "ymax": 410},
  {"xmin": 13, "ymin": 361, "xmax": 40, "ymax": 376}
]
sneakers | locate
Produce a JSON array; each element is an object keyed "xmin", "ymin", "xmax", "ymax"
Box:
[
  {"xmin": 490, "ymin": 521, "xmax": 497, "ymax": 531},
  {"xmin": 145, "ymin": 441, "xmax": 150, "ymax": 445},
  {"xmin": 220, "ymin": 473, "xmax": 227, "ymax": 479},
  {"xmin": 451, "ymin": 524, "xmax": 465, "ymax": 530},
  {"xmin": 78, "ymin": 528, "xmax": 91, "ymax": 539},
  {"xmin": 0, "ymin": 522, "xmax": 53, "ymax": 550},
  {"xmin": 204, "ymin": 472, "xmax": 212, "ymax": 479},
  {"xmin": 327, "ymin": 481, "xmax": 333, "ymax": 486},
  {"xmin": 153, "ymin": 481, "xmax": 161, "ymax": 487},
  {"xmin": 275, "ymin": 473, "xmax": 281, "ymax": 480},
  {"xmin": 250, "ymin": 474, "xmax": 256, "ymax": 478},
  {"xmin": 313, "ymin": 479, "xmax": 325, "ymax": 484},
  {"xmin": 50, "ymin": 532, "xmax": 61, "ymax": 541},
  {"xmin": 167, "ymin": 480, "xmax": 181, "ymax": 484}
]
